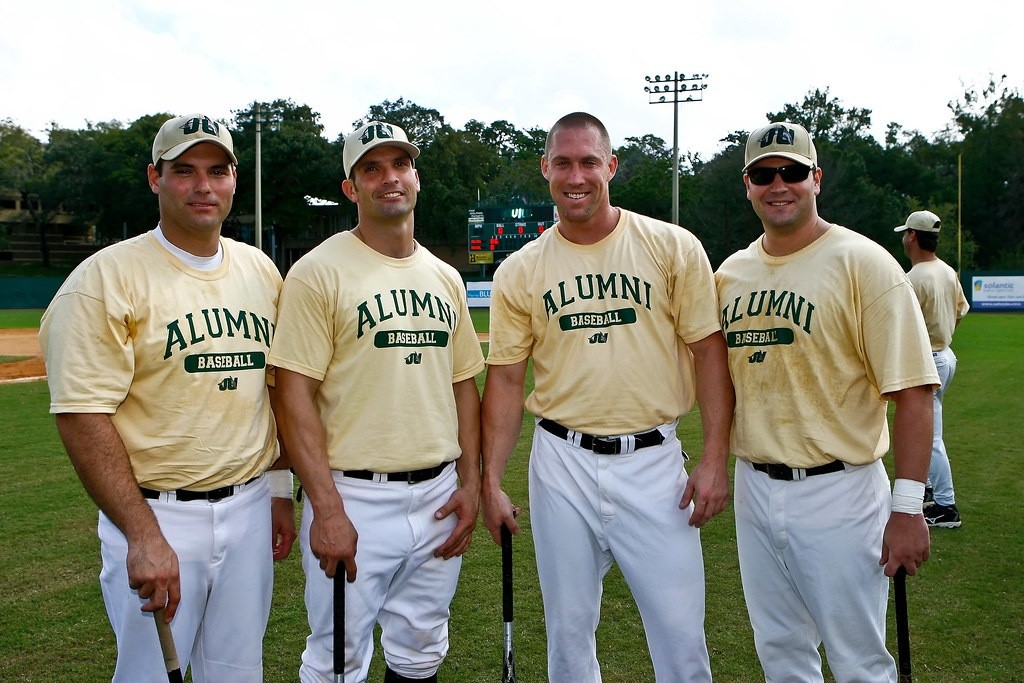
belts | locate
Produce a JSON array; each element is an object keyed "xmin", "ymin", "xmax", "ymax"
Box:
[
  {"xmin": 140, "ymin": 477, "xmax": 259, "ymax": 503},
  {"xmin": 343, "ymin": 461, "xmax": 453, "ymax": 485},
  {"xmin": 539, "ymin": 419, "xmax": 665, "ymax": 454},
  {"xmin": 745, "ymin": 459, "xmax": 845, "ymax": 482}
]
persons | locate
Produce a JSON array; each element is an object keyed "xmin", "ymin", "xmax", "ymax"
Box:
[
  {"xmin": 37, "ymin": 114, "xmax": 297, "ymax": 683},
  {"xmin": 714, "ymin": 123, "xmax": 941, "ymax": 683},
  {"xmin": 267, "ymin": 121, "xmax": 485, "ymax": 683},
  {"xmin": 479, "ymin": 113, "xmax": 735, "ymax": 683},
  {"xmin": 894, "ymin": 210, "xmax": 970, "ymax": 527}
]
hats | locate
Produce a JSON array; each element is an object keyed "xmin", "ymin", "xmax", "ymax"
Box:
[
  {"xmin": 892, "ymin": 210, "xmax": 942, "ymax": 233},
  {"xmin": 343, "ymin": 121, "xmax": 420, "ymax": 179},
  {"xmin": 742, "ymin": 121, "xmax": 817, "ymax": 170},
  {"xmin": 152, "ymin": 113, "xmax": 239, "ymax": 166}
]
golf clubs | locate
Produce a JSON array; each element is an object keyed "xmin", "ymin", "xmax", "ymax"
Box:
[
  {"xmin": 155, "ymin": 610, "xmax": 182, "ymax": 683},
  {"xmin": 501, "ymin": 524, "xmax": 516, "ymax": 681},
  {"xmin": 332, "ymin": 569, "xmax": 345, "ymax": 683},
  {"xmin": 895, "ymin": 569, "xmax": 912, "ymax": 682}
]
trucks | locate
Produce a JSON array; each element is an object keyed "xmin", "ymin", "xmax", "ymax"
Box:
[{"xmin": 468, "ymin": 196, "xmax": 557, "ymax": 280}]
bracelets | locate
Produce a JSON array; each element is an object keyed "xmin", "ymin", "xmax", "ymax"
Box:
[
  {"xmin": 890, "ymin": 478, "xmax": 926, "ymax": 515},
  {"xmin": 265, "ymin": 469, "xmax": 294, "ymax": 499}
]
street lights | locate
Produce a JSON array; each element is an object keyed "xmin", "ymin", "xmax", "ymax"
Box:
[
  {"xmin": 644, "ymin": 73, "xmax": 709, "ymax": 225},
  {"xmin": 234, "ymin": 107, "xmax": 284, "ymax": 248}
]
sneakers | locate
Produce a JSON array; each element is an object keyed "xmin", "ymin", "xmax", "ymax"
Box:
[
  {"xmin": 922, "ymin": 501, "xmax": 962, "ymax": 529},
  {"xmin": 921, "ymin": 487, "xmax": 936, "ymax": 509}
]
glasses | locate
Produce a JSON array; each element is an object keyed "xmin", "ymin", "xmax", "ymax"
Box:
[{"xmin": 747, "ymin": 165, "xmax": 815, "ymax": 186}]
